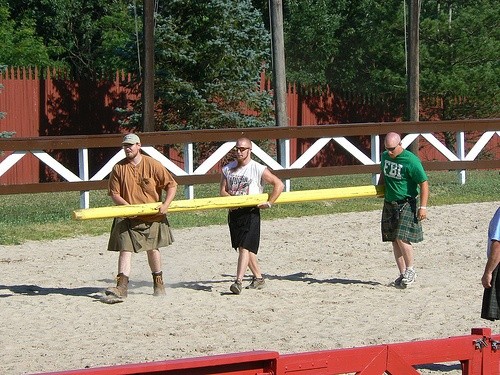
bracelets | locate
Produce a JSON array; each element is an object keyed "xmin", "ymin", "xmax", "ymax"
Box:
[
  {"xmin": 268, "ymin": 201, "xmax": 271, "ymax": 208},
  {"xmin": 418, "ymin": 207, "xmax": 426, "ymax": 210}
]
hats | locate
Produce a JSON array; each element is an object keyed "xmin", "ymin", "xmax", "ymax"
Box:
[{"xmin": 122, "ymin": 134, "xmax": 140, "ymax": 144}]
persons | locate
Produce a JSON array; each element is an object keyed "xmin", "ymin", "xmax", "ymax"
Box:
[
  {"xmin": 221, "ymin": 137, "xmax": 287, "ymax": 294},
  {"xmin": 375, "ymin": 132, "xmax": 428, "ymax": 287},
  {"xmin": 105, "ymin": 134, "xmax": 177, "ymax": 297},
  {"xmin": 480, "ymin": 205, "xmax": 500, "ymax": 320}
]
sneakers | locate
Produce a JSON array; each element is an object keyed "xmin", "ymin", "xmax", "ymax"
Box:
[
  {"xmin": 394, "ymin": 274, "xmax": 405, "ymax": 284},
  {"xmin": 230, "ymin": 278, "xmax": 242, "ymax": 294},
  {"xmin": 402, "ymin": 268, "xmax": 415, "ymax": 284},
  {"xmin": 245, "ymin": 275, "xmax": 267, "ymax": 290}
]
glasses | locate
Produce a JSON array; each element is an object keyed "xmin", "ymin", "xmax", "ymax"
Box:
[
  {"xmin": 385, "ymin": 141, "xmax": 401, "ymax": 151},
  {"xmin": 233, "ymin": 146, "xmax": 252, "ymax": 152}
]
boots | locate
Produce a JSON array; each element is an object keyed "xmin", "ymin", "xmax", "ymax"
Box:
[
  {"xmin": 105, "ymin": 273, "xmax": 129, "ymax": 299},
  {"xmin": 152, "ymin": 269, "xmax": 166, "ymax": 296}
]
